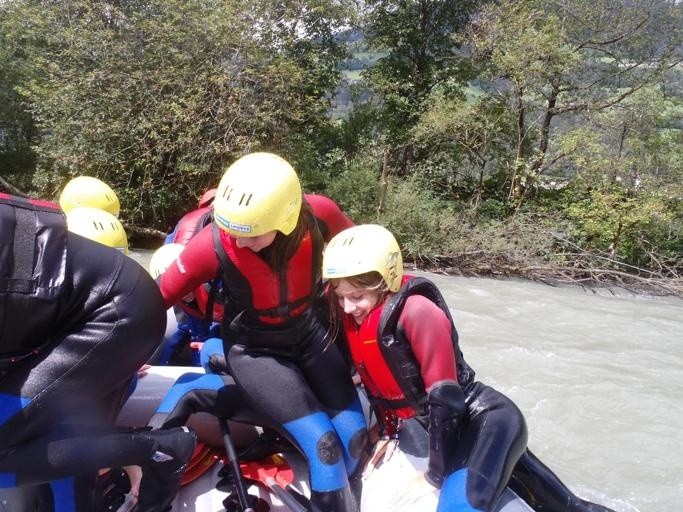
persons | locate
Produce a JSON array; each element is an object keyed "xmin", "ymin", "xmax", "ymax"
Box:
[
  {"xmin": 0, "ymin": 191, "xmax": 195, "ymax": 512},
  {"xmin": 152, "ymin": 153, "xmax": 371, "ymax": 512},
  {"xmin": 58, "ymin": 176, "xmax": 129, "ymax": 257},
  {"xmin": 158, "ymin": 187, "xmax": 224, "ymax": 352},
  {"xmin": 322, "ymin": 223, "xmax": 614, "ymax": 512}
]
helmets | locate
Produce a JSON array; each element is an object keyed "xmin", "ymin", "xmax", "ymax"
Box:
[
  {"xmin": 321, "ymin": 224, "xmax": 404, "ymax": 294},
  {"xmin": 59, "ymin": 176, "xmax": 130, "ymax": 256},
  {"xmin": 213, "ymin": 151, "xmax": 304, "ymax": 239}
]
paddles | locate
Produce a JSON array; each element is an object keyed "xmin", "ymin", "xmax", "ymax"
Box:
[
  {"xmin": 175, "ymin": 450, "xmax": 221, "ymax": 487},
  {"xmin": 218, "ymin": 446, "xmax": 301, "ymax": 508},
  {"xmin": 176, "ymin": 443, "xmax": 214, "ymax": 474},
  {"xmin": 269, "ymin": 452, "xmax": 316, "ymax": 507}
]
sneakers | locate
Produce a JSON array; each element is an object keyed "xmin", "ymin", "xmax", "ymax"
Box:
[{"xmin": 136, "ymin": 424, "xmax": 198, "ymax": 511}]
